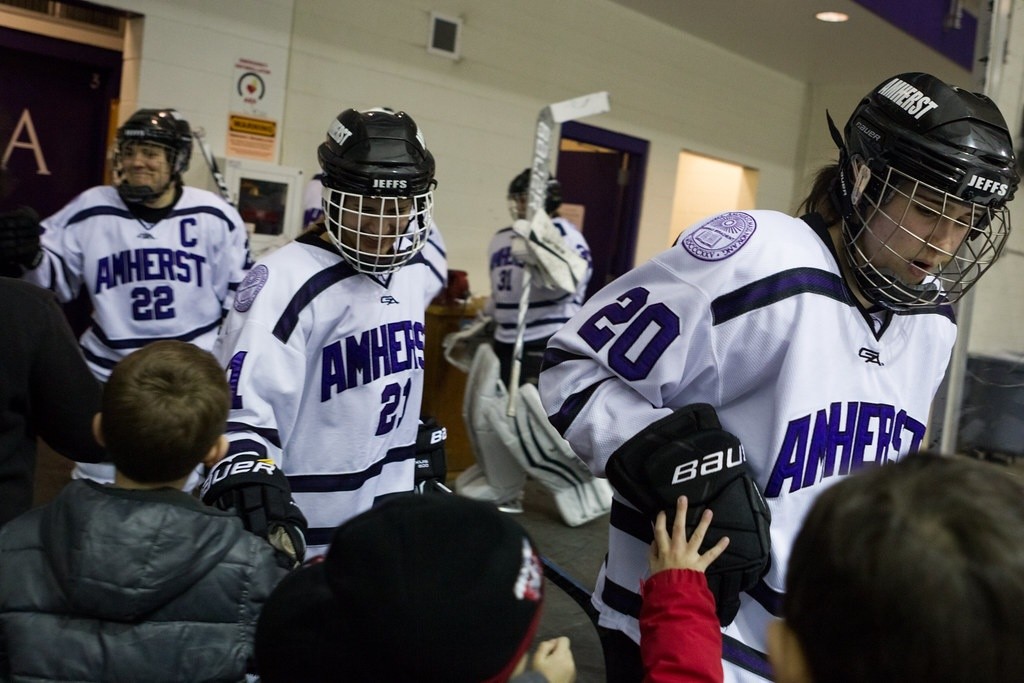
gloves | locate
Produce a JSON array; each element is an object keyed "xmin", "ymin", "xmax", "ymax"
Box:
[
  {"xmin": 604, "ymin": 403, "xmax": 772, "ymax": 596},
  {"xmin": 198, "ymin": 450, "xmax": 293, "ymax": 542}
]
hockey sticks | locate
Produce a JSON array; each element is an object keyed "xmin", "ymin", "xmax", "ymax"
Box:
[
  {"xmin": 195, "ymin": 123, "xmax": 239, "ymax": 221},
  {"xmin": 502, "ymin": 88, "xmax": 612, "ymax": 417}
]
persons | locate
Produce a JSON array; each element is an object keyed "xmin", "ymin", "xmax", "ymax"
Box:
[
  {"xmin": 251, "ymin": 484, "xmax": 578, "ymax": 683},
  {"xmin": 1, "ymin": 285, "xmax": 308, "ymax": 683},
  {"xmin": 638, "ymin": 448, "xmax": 1024, "ymax": 683},
  {"xmin": 457, "ymin": 163, "xmax": 613, "ymax": 526},
  {"xmin": 535, "ymin": 71, "xmax": 1024, "ymax": 683},
  {"xmin": 199, "ymin": 104, "xmax": 449, "ymax": 566},
  {"xmin": 0, "ymin": 103, "xmax": 251, "ymax": 523}
]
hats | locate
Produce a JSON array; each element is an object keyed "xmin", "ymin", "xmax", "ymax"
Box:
[{"xmin": 254, "ymin": 489, "xmax": 549, "ymax": 683}]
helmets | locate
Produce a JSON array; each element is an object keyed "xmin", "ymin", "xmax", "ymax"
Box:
[
  {"xmin": 838, "ymin": 71, "xmax": 1021, "ymax": 316},
  {"xmin": 310, "ymin": 105, "xmax": 440, "ymax": 276},
  {"xmin": 115, "ymin": 105, "xmax": 193, "ymax": 206},
  {"xmin": 507, "ymin": 167, "xmax": 564, "ymax": 224}
]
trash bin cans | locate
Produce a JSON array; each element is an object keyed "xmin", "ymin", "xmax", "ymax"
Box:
[{"xmin": 957, "ymin": 349, "xmax": 1024, "ymax": 457}]
